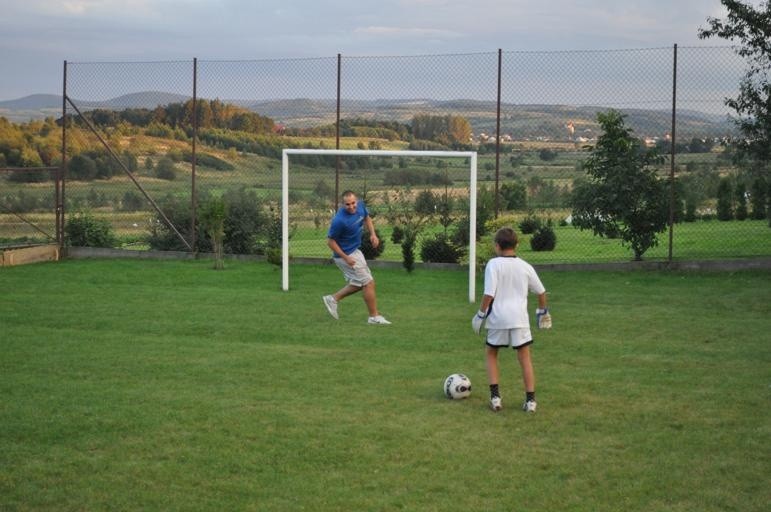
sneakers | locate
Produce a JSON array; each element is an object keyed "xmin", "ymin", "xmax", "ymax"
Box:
[
  {"xmin": 322, "ymin": 295, "xmax": 339, "ymax": 320},
  {"xmin": 368, "ymin": 316, "xmax": 392, "ymax": 325},
  {"xmin": 489, "ymin": 397, "xmax": 502, "ymax": 411},
  {"xmin": 523, "ymin": 401, "xmax": 537, "ymax": 412}
]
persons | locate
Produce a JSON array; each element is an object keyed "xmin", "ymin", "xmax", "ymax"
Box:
[
  {"xmin": 472, "ymin": 228, "xmax": 552, "ymax": 413},
  {"xmin": 322, "ymin": 191, "xmax": 392, "ymax": 324}
]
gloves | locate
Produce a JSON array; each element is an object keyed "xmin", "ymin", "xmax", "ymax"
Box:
[
  {"xmin": 536, "ymin": 309, "xmax": 551, "ymax": 329},
  {"xmin": 472, "ymin": 310, "xmax": 484, "ymax": 335}
]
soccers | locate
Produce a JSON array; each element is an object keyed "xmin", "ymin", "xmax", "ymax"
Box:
[{"xmin": 443, "ymin": 374, "xmax": 471, "ymax": 400}]
